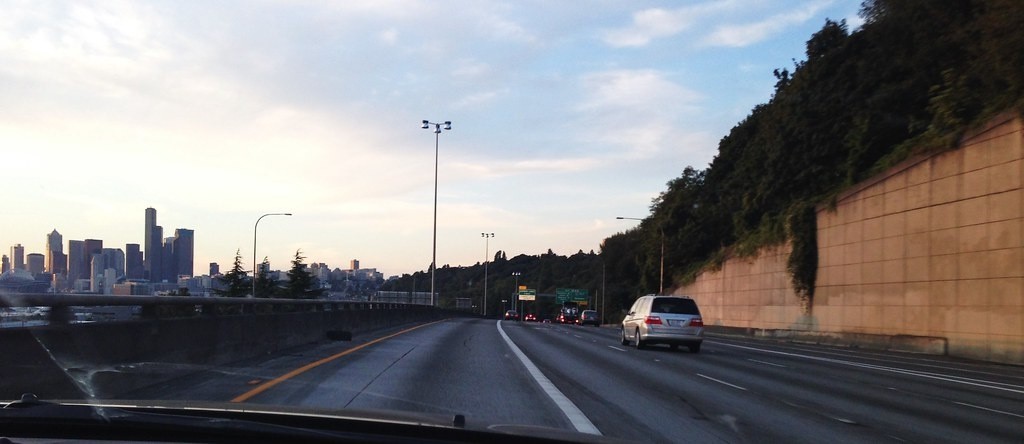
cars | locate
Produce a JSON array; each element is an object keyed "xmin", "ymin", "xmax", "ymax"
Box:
[
  {"xmin": 525, "ymin": 305, "xmax": 578, "ymax": 326},
  {"xmin": 504, "ymin": 310, "xmax": 520, "ymax": 321}
]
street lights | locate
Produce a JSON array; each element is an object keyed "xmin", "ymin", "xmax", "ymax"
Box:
[
  {"xmin": 481, "ymin": 232, "xmax": 494, "ymax": 317},
  {"xmin": 512, "ymin": 271, "xmax": 521, "ymax": 312},
  {"xmin": 251, "ymin": 213, "xmax": 294, "ymax": 298},
  {"xmin": 422, "ymin": 120, "xmax": 452, "ymax": 307},
  {"xmin": 617, "ymin": 216, "xmax": 665, "ymax": 296}
]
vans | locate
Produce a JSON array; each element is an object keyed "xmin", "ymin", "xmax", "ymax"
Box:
[{"xmin": 621, "ymin": 295, "xmax": 705, "ymax": 352}]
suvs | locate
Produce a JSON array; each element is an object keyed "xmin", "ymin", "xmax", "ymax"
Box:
[{"xmin": 578, "ymin": 309, "xmax": 600, "ymax": 328}]
